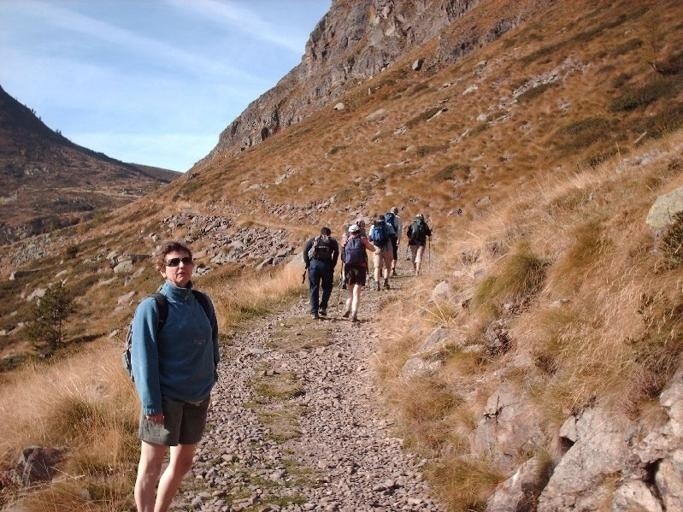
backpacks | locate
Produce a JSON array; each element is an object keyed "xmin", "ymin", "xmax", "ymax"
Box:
[
  {"xmin": 344, "ymin": 235, "xmax": 366, "ymax": 263},
  {"xmin": 122, "ymin": 290, "xmax": 218, "ymax": 382},
  {"xmin": 313, "ymin": 235, "xmax": 334, "ymax": 260},
  {"xmin": 411, "ymin": 219, "xmax": 425, "ymax": 243},
  {"xmin": 384, "ymin": 213, "xmax": 397, "ymax": 228},
  {"xmin": 371, "ymin": 220, "xmax": 387, "ymax": 246}
]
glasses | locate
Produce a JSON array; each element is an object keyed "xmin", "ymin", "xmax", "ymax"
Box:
[{"xmin": 164, "ymin": 257, "xmax": 191, "ymax": 265}]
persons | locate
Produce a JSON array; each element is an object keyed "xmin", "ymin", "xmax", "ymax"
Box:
[
  {"xmin": 368, "ymin": 215, "xmax": 396, "ymax": 292},
  {"xmin": 406, "ymin": 213, "xmax": 432, "ymax": 276},
  {"xmin": 130, "ymin": 239, "xmax": 220, "ymax": 512},
  {"xmin": 340, "ymin": 225, "xmax": 376, "ymax": 322},
  {"xmin": 384, "ymin": 207, "xmax": 402, "ymax": 276},
  {"xmin": 339, "ymin": 219, "xmax": 366, "ymax": 289},
  {"xmin": 303, "ymin": 226, "xmax": 339, "ymax": 320}
]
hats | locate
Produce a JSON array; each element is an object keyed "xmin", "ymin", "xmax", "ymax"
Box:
[
  {"xmin": 416, "ymin": 213, "xmax": 423, "ymax": 219},
  {"xmin": 390, "ymin": 207, "xmax": 398, "ymax": 213},
  {"xmin": 348, "ymin": 225, "xmax": 360, "ymax": 232}
]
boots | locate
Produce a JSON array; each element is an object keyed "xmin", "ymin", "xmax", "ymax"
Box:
[
  {"xmin": 383, "ymin": 279, "xmax": 390, "ymax": 288},
  {"xmin": 374, "ymin": 281, "xmax": 380, "ymax": 291}
]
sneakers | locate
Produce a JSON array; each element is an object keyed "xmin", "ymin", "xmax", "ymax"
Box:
[
  {"xmin": 351, "ymin": 317, "xmax": 360, "ymax": 322},
  {"xmin": 410, "ymin": 267, "xmax": 420, "ymax": 276},
  {"xmin": 391, "ymin": 272, "xmax": 397, "ymax": 276},
  {"xmin": 343, "ymin": 309, "xmax": 350, "ymax": 317},
  {"xmin": 319, "ymin": 307, "xmax": 327, "ymax": 315},
  {"xmin": 311, "ymin": 314, "xmax": 318, "ymax": 319}
]
